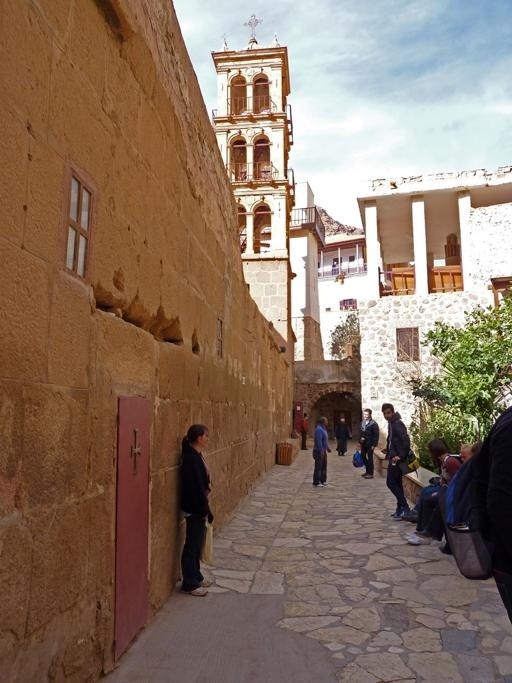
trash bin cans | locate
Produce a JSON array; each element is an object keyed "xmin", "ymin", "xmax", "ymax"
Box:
[
  {"xmin": 291, "ymin": 432, "xmax": 302, "ymax": 438},
  {"xmin": 277, "ymin": 442, "xmax": 292, "ymax": 464}
]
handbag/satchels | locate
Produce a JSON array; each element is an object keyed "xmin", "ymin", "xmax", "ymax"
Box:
[
  {"xmin": 201, "ymin": 519, "xmax": 214, "ymax": 566},
  {"xmin": 352, "ymin": 450, "xmax": 365, "ymax": 467},
  {"xmin": 398, "ymin": 450, "xmax": 419, "ymax": 475}
]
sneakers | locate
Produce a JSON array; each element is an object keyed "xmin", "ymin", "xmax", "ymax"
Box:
[
  {"xmin": 312, "ymin": 482, "xmax": 328, "ymax": 487},
  {"xmin": 362, "ymin": 471, "xmax": 372, "ymax": 479},
  {"xmin": 391, "ymin": 509, "xmax": 432, "ymax": 545},
  {"xmin": 181, "ymin": 576, "xmax": 213, "ymax": 596}
]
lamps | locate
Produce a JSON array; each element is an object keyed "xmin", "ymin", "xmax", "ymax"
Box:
[{"xmin": 390, "ymin": 179, "xmax": 397, "ymax": 188}]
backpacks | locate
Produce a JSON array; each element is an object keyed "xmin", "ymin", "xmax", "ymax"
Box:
[{"xmin": 439, "ymin": 405, "xmax": 511, "ymax": 580}]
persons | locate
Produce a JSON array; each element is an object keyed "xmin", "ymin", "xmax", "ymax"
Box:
[
  {"xmin": 311, "ymin": 416, "xmax": 332, "ymax": 487},
  {"xmin": 475, "ymin": 398, "xmax": 510, "ymax": 623},
  {"xmin": 359, "ymin": 408, "xmax": 379, "ymax": 478},
  {"xmin": 399, "ymin": 436, "xmax": 485, "ymax": 554},
  {"xmin": 379, "ymin": 403, "xmax": 412, "ymax": 520},
  {"xmin": 334, "ymin": 416, "xmax": 353, "ymax": 455},
  {"xmin": 174, "ymin": 423, "xmax": 215, "ymax": 597},
  {"xmin": 299, "ymin": 412, "xmax": 310, "ymax": 450}
]
